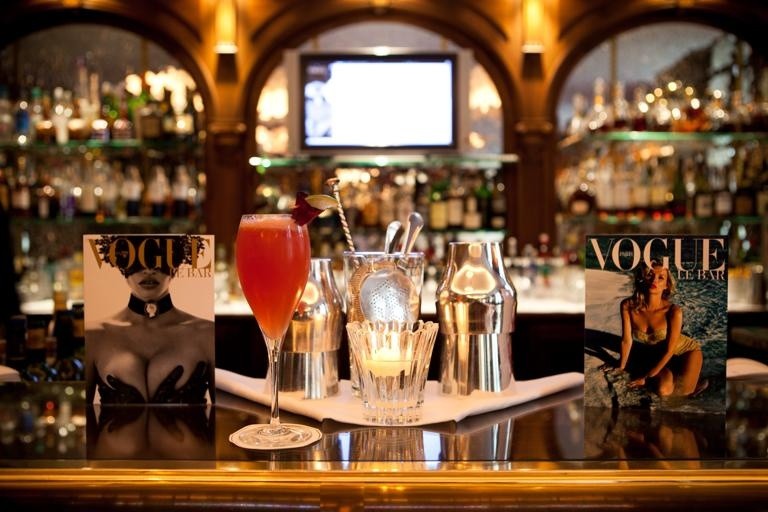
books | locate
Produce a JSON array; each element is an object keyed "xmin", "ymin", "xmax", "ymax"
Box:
[{"xmin": 583, "ymin": 231, "xmax": 730, "ymax": 417}]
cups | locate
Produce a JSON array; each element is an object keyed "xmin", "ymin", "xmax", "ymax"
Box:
[{"xmin": 345, "ymin": 251, "xmax": 438, "ymax": 409}]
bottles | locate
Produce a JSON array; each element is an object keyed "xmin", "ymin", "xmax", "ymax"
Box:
[
  {"xmin": 430, "ymin": 167, "xmax": 493, "ymax": 232},
  {"xmin": 279, "ymin": 259, "xmax": 341, "ymax": 400},
  {"xmin": 443, "ymin": 240, "xmax": 515, "ymax": 392}
]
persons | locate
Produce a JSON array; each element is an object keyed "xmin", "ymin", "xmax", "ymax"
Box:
[{"xmin": 604, "ymin": 257, "xmax": 706, "ymax": 404}]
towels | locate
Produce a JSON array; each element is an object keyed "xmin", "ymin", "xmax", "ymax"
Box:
[{"xmin": 215, "ymin": 368, "xmax": 585, "ymax": 428}]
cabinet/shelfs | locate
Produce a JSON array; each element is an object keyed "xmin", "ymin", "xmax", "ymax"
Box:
[
  {"xmin": 556, "ymin": 130, "xmax": 768, "ymax": 225},
  {"xmin": 250, "ymin": 156, "xmax": 509, "ymax": 241},
  {"xmin": 0, "ymin": 138, "xmax": 203, "ymax": 227}
]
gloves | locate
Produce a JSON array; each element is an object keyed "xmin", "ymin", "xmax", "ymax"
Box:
[
  {"xmin": 96, "ymin": 372, "xmax": 146, "ymax": 405},
  {"xmin": 96, "ymin": 405, "xmax": 146, "ymax": 433},
  {"xmin": 152, "ymin": 404, "xmax": 210, "ymax": 442},
  {"xmin": 152, "ymin": 360, "xmax": 210, "ymax": 404}
]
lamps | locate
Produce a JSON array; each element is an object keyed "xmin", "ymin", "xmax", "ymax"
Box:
[
  {"xmin": 521, "ymin": 0, "xmax": 544, "ymax": 53},
  {"xmin": 215, "ymin": 0, "xmax": 238, "ymax": 54}
]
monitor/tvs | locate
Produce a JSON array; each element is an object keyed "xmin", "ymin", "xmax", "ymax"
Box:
[{"xmin": 299, "ymin": 51, "xmax": 459, "ymax": 150}]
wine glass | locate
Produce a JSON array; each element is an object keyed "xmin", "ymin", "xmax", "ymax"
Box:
[{"xmin": 234, "ymin": 212, "xmax": 313, "ymax": 445}]
